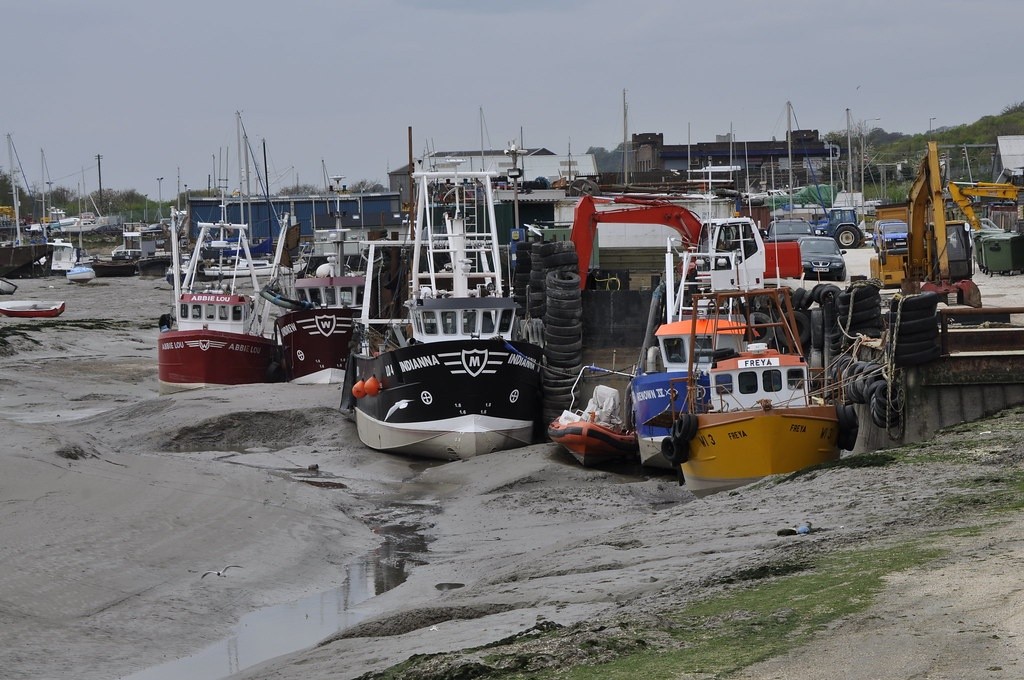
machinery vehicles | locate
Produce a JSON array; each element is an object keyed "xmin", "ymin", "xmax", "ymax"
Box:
[
  {"xmin": 870, "ymin": 142, "xmax": 1019, "ymax": 308},
  {"xmin": 791, "ymin": 105, "xmax": 873, "ymax": 249}
]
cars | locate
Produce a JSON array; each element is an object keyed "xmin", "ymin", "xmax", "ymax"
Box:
[
  {"xmin": 96, "ymin": 225, "xmax": 123, "ymax": 230},
  {"xmin": 111, "ymin": 245, "xmax": 123, "ymax": 257},
  {"xmin": 149, "ymin": 224, "xmax": 166, "ymax": 229},
  {"xmin": 793, "ymin": 237, "xmax": 847, "ymax": 282},
  {"xmin": 81, "ymin": 212, "xmax": 96, "ymax": 224}
]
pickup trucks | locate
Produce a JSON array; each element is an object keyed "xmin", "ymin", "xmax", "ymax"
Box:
[{"xmin": 872, "ymin": 219, "xmax": 909, "ymax": 254}]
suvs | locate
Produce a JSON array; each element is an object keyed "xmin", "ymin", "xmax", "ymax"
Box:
[{"xmin": 757, "ymin": 217, "xmax": 816, "ymax": 243}]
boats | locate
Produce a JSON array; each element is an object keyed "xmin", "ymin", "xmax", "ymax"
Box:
[
  {"xmin": 203, "ymin": 258, "xmax": 307, "ymax": 276},
  {"xmin": 92, "ymin": 256, "xmax": 172, "ymax": 278},
  {"xmin": 200, "ymin": 110, "xmax": 274, "ymax": 260},
  {"xmin": 157, "ymin": 146, "xmax": 282, "ymax": 387},
  {"xmin": 60, "ymin": 220, "xmax": 98, "ymax": 232},
  {"xmin": 338, "ymin": 158, "xmax": 545, "ymax": 460},
  {"xmin": 274, "ymin": 174, "xmax": 364, "ymax": 385},
  {"xmin": 0, "ymin": 300, "xmax": 66, "ymax": 317},
  {"xmin": 547, "ymin": 365, "xmax": 638, "ymax": 467},
  {"xmin": 66, "ymin": 267, "xmax": 95, "ymax": 284},
  {"xmin": 629, "ymin": 236, "xmax": 859, "ymax": 499}
]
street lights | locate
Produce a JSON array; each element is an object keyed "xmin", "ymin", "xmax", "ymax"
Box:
[
  {"xmin": 157, "ymin": 178, "xmax": 164, "ymax": 211},
  {"xmin": 861, "ymin": 117, "xmax": 880, "ymax": 233},
  {"xmin": 503, "ymin": 139, "xmax": 527, "ymax": 229},
  {"xmin": 929, "ymin": 118, "xmax": 936, "ymax": 141}
]
